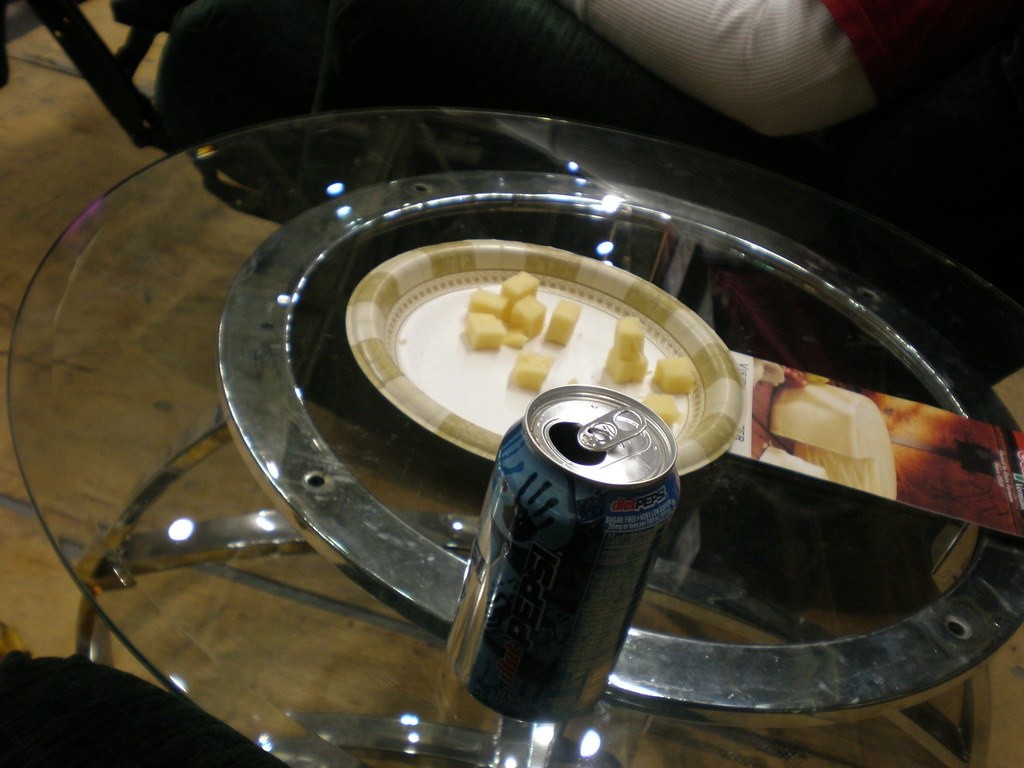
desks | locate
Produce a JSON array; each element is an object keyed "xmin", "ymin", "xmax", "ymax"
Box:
[{"xmin": 9, "ymin": 108, "xmax": 1023, "ymax": 768}]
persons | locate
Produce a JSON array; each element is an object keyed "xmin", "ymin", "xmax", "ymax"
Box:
[
  {"xmin": 0, "ymin": 651, "xmax": 289, "ymax": 767},
  {"xmin": 158, "ymin": 0, "xmax": 1013, "ymax": 147}
]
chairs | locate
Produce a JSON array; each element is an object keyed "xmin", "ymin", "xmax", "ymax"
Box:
[{"xmin": 0, "ymin": 0, "xmax": 1024, "ymax": 614}]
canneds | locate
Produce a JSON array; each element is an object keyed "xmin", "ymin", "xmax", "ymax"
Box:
[{"xmin": 447, "ymin": 383, "xmax": 681, "ymax": 723}]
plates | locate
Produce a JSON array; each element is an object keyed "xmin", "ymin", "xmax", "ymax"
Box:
[{"xmin": 344, "ymin": 237, "xmax": 745, "ymax": 477}]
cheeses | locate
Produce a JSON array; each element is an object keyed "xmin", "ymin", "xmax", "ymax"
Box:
[
  {"xmin": 464, "ymin": 269, "xmax": 694, "ymax": 423},
  {"xmin": 769, "ymin": 380, "xmax": 898, "ymax": 501}
]
